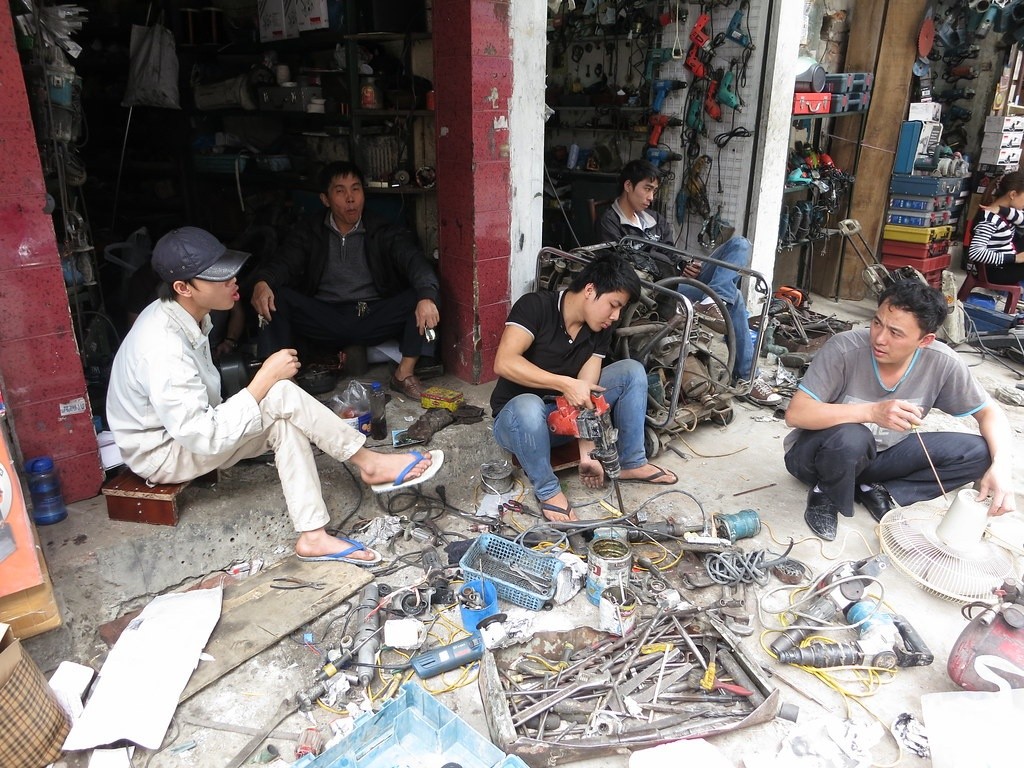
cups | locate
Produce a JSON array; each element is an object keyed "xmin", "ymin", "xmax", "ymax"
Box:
[
  {"xmin": 276, "ymin": 64, "xmax": 290, "ymax": 86},
  {"xmin": 457, "ymin": 580, "xmax": 499, "ymax": 633},
  {"xmin": 360, "ymin": 71, "xmax": 383, "ymax": 110}
]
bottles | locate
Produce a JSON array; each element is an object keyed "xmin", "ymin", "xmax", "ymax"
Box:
[
  {"xmin": 567, "ymin": 142, "xmax": 580, "ymax": 170},
  {"xmin": 26, "ymin": 456, "xmax": 67, "ymax": 526},
  {"xmin": 370, "ymin": 382, "xmax": 388, "ymax": 441}
]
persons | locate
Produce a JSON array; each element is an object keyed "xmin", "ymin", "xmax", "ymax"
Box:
[
  {"xmin": 251, "ymin": 164, "xmax": 441, "ymax": 400},
  {"xmin": 105, "ymin": 228, "xmax": 444, "ymax": 565},
  {"xmin": 784, "ymin": 281, "xmax": 1024, "ymax": 540},
  {"xmin": 968, "ymin": 170, "xmax": 1024, "ymax": 283},
  {"xmin": 126, "ymin": 225, "xmax": 245, "ymax": 358},
  {"xmin": 490, "ymin": 256, "xmax": 678, "ymax": 522},
  {"xmin": 597, "ymin": 158, "xmax": 782, "ymax": 405}
]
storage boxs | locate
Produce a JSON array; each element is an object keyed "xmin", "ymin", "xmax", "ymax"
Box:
[
  {"xmin": 551, "ymin": 199, "xmax": 572, "ymax": 209},
  {"xmin": 792, "ymin": 72, "xmax": 874, "ymax": 114},
  {"xmin": 964, "ymin": 115, "xmax": 1024, "ymax": 246},
  {"xmin": 747, "ymin": 310, "xmax": 853, "ymax": 352},
  {"xmin": 421, "ymin": 386, "xmax": 464, "ymax": 412},
  {"xmin": 257, "ymin": 87, "xmax": 322, "ymax": 112},
  {"xmin": 554, "ymin": 107, "xmax": 648, "ymax": 127},
  {"xmin": 257, "ymin": 0, "xmax": 329, "ymax": 42}
]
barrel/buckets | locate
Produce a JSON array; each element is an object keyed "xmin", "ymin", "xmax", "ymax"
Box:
[{"xmin": 586, "ymin": 535, "xmax": 637, "ymax": 635}]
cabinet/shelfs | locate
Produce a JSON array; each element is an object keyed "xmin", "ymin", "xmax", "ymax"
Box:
[
  {"xmin": 174, "ymin": 0, "xmax": 437, "ymax": 195},
  {"xmin": 546, "ymin": 0, "xmax": 663, "ymax": 210},
  {"xmin": 11, "ymin": 0, "xmax": 113, "ymax": 410},
  {"xmin": 777, "ymin": 110, "xmax": 868, "ymax": 304}
]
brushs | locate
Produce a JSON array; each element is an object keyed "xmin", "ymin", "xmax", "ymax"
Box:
[{"xmin": 688, "ymin": 669, "xmax": 754, "ymax": 697}]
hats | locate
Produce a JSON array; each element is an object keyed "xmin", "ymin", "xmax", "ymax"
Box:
[{"xmin": 151, "ymin": 225, "xmax": 251, "ymax": 281}]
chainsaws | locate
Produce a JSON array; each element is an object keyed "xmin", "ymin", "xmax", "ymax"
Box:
[
  {"xmin": 412, "ymin": 612, "xmax": 511, "ymax": 679},
  {"xmin": 914, "ymin": 143, "xmax": 970, "ymax": 179}
]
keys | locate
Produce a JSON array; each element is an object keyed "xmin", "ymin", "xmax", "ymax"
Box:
[
  {"xmin": 356, "ymin": 304, "xmax": 368, "ymax": 316},
  {"xmin": 258, "ymin": 314, "xmax": 266, "ymax": 327}
]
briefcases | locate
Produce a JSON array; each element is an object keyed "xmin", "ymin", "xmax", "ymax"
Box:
[{"xmin": 792, "ymin": 93, "xmax": 831, "ymax": 115}]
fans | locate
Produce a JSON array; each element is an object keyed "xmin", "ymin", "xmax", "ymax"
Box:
[{"xmin": 876, "ymin": 488, "xmax": 1024, "ymax": 609}]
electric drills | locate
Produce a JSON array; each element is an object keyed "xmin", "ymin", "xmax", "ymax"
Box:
[
  {"xmin": 689, "ymin": 10, "xmax": 755, "ymax": 57},
  {"xmin": 778, "ymin": 598, "xmax": 934, "ymax": 672},
  {"xmin": 548, "ymin": 392, "xmax": 627, "ymax": 516},
  {"xmin": 687, "ymin": 96, "xmax": 709, "ymax": 138},
  {"xmin": 716, "ymin": 72, "xmax": 743, "ymax": 113},
  {"xmin": 770, "ymin": 552, "xmax": 890, "ymax": 655},
  {"xmin": 684, "ymin": 42, "xmax": 712, "ymax": 82},
  {"xmin": 652, "ymin": 79, "xmax": 688, "ymax": 113},
  {"xmin": 649, "ymin": 114, "xmax": 683, "ymax": 147},
  {"xmin": 646, "ymin": 48, "xmax": 683, "ymax": 79},
  {"xmin": 704, "ymin": 79, "xmax": 722, "ymax": 122},
  {"xmin": 646, "ymin": 148, "xmax": 682, "ymax": 171}
]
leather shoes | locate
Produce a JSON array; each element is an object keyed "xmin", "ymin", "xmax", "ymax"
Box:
[
  {"xmin": 804, "ymin": 483, "xmax": 838, "ymax": 541},
  {"xmin": 854, "ymin": 484, "xmax": 896, "ymax": 524}
]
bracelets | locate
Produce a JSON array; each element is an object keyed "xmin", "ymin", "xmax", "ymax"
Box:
[{"xmin": 225, "ymin": 338, "xmax": 239, "ymax": 351}]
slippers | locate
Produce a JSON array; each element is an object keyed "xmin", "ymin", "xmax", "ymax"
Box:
[
  {"xmin": 618, "ymin": 462, "xmax": 678, "ymax": 484},
  {"xmin": 371, "ymin": 449, "xmax": 444, "ymax": 492},
  {"xmin": 535, "ymin": 493, "xmax": 577, "ymax": 531},
  {"xmin": 296, "ymin": 537, "xmax": 382, "ymax": 566}
]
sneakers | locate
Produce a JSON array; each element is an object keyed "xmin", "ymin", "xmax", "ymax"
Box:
[
  {"xmin": 749, "ymin": 378, "xmax": 783, "ymax": 405},
  {"xmin": 692, "ymin": 301, "xmax": 727, "ymax": 335}
]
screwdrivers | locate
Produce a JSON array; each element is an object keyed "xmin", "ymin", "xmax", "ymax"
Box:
[
  {"xmin": 316, "ymin": 625, "xmax": 383, "ymax": 682},
  {"xmin": 554, "ymin": 643, "xmax": 573, "ymax": 689}
]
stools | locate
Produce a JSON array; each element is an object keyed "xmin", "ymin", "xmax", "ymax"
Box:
[
  {"xmin": 102, "ymin": 466, "xmax": 221, "ymax": 526},
  {"xmin": 512, "ymin": 433, "xmax": 580, "ymax": 473}
]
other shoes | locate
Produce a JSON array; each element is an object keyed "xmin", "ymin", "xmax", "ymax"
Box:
[{"xmin": 390, "ymin": 371, "xmax": 426, "ymax": 401}]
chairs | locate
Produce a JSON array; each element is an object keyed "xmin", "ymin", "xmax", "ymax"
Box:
[
  {"xmin": 957, "ymin": 261, "xmax": 1021, "ymax": 315},
  {"xmin": 588, "ymin": 197, "xmax": 616, "ymax": 237}
]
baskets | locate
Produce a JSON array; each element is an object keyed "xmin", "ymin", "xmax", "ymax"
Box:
[{"xmin": 458, "ymin": 532, "xmax": 562, "ymax": 612}]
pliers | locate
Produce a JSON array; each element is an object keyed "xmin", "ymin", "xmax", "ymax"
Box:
[
  {"xmin": 517, "ymin": 653, "xmax": 561, "ymax": 676},
  {"xmin": 502, "ymin": 500, "xmax": 542, "ymax": 519},
  {"xmin": 270, "ymin": 577, "xmax": 326, "ymax": 590}
]
